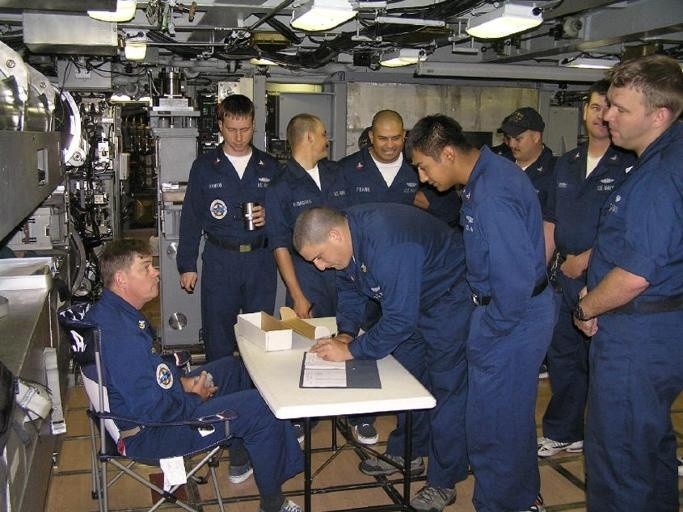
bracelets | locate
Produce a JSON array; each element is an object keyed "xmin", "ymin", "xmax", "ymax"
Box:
[{"xmin": 572, "ymin": 298, "xmax": 591, "ymax": 321}]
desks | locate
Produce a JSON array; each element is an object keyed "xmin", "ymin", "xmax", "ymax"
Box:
[{"xmin": 223, "ymin": 316, "xmax": 439, "ymax": 512}]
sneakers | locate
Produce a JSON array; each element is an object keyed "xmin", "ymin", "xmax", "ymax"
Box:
[
  {"xmin": 291, "ymin": 422, "xmax": 305, "ymax": 444},
  {"xmin": 538, "ymin": 364, "xmax": 551, "ymax": 381},
  {"xmin": 353, "ymin": 420, "xmax": 379, "ymax": 445},
  {"xmin": 357, "ymin": 451, "xmax": 426, "ymax": 476},
  {"xmin": 257, "ymin": 497, "xmax": 304, "ymax": 512},
  {"xmin": 536, "ymin": 434, "xmax": 583, "ymax": 458},
  {"xmin": 406, "ymin": 481, "xmax": 457, "ymax": 511},
  {"xmin": 227, "ymin": 460, "xmax": 253, "ymax": 484}
]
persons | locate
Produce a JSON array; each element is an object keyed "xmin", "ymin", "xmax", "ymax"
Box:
[
  {"xmin": 489, "ymin": 115, "xmax": 516, "ymax": 164},
  {"xmin": 79, "ymin": 234, "xmax": 310, "ymax": 510},
  {"xmin": 402, "ymin": 114, "xmax": 561, "ymax": 511},
  {"xmin": 411, "ymin": 186, "xmax": 462, "ymax": 232},
  {"xmin": 329, "ymin": 109, "xmax": 421, "ymax": 204},
  {"xmin": 263, "ymin": 111, "xmax": 381, "ymax": 446},
  {"xmin": 530, "ymin": 80, "xmax": 639, "ymax": 463},
  {"xmin": 290, "ymin": 200, "xmax": 474, "ymax": 511},
  {"xmin": 569, "ymin": 51, "xmax": 682, "ymax": 512},
  {"xmin": 174, "ymin": 95, "xmax": 283, "ymax": 367},
  {"xmin": 490, "ymin": 106, "xmax": 554, "ymax": 381}
]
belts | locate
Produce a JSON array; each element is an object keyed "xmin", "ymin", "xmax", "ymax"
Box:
[
  {"xmin": 471, "ymin": 271, "xmax": 551, "ymax": 311},
  {"xmin": 604, "ymin": 292, "xmax": 682, "ymax": 315},
  {"xmin": 203, "ymin": 230, "xmax": 269, "ymax": 254}
]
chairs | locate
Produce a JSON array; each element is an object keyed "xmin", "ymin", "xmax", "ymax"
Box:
[{"xmin": 57, "ymin": 305, "xmax": 239, "ymax": 512}]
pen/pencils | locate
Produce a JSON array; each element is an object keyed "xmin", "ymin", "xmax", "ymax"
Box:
[
  {"xmin": 332, "ymin": 333, "xmax": 336, "ymax": 339},
  {"xmin": 308, "ymin": 302, "xmax": 314, "ymax": 315}
]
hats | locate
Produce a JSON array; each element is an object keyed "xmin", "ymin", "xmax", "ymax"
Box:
[{"xmin": 495, "ymin": 106, "xmax": 544, "ymax": 135}]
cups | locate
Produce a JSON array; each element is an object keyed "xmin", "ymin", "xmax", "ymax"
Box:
[{"xmin": 241, "ymin": 200, "xmax": 262, "ymax": 232}]
